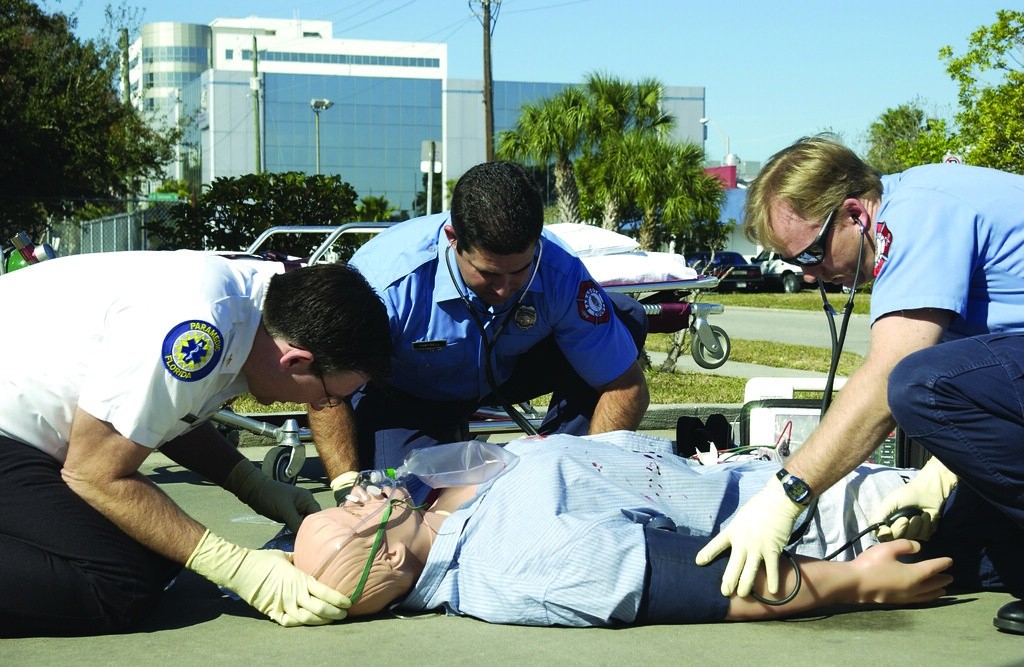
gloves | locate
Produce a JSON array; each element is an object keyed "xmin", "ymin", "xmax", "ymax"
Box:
[
  {"xmin": 868, "ymin": 456, "xmax": 958, "ymax": 542},
  {"xmin": 185, "ymin": 528, "xmax": 352, "ymax": 627},
  {"xmin": 330, "ymin": 471, "xmax": 359, "ymax": 507},
  {"xmin": 695, "ymin": 476, "xmax": 809, "ymax": 597}
]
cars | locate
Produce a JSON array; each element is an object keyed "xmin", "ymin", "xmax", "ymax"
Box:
[
  {"xmin": 750, "ymin": 248, "xmax": 844, "ymax": 295},
  {"xmin": 685, "ymin": 250, "xmax": 763, "ymax": 292}
]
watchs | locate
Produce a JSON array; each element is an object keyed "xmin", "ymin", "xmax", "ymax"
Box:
[{"xmin": 775, "ymin": 467, "xmax": 817, "ymax": 507}]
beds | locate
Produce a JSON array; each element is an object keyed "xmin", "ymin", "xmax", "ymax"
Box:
[{"xmin": 211, "ymin": 222, "xmax": 731, "ymax": 487}]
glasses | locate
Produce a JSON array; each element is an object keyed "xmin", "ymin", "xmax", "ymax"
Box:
[
  {"xmin": 288, "ymin": 343, "xmax": 341, "ymax": 408},
  {"xmin": 781, "ymin": 193, "xmax": 855, "ymax": 266}
]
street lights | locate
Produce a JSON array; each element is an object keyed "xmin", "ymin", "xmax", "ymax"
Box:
[{"xmin": 310, "ymin": 97, "xmax": 334, "ymax": 175}]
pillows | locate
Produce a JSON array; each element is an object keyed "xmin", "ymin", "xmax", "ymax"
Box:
[
  {"xmin": 580, "ymin": 250, "xmax": 699, "ymax": 286},
  {"xmin": 550, "ymin": 221, "xmax": 642, "ymax": 257}
]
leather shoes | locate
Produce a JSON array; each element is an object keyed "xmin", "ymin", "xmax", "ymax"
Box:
[{"xmin": 993, "ymin": 600, "xmax": 1024, "ymax": 632}]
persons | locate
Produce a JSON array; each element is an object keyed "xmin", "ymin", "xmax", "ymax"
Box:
[
  {"xmin": 0, "ymin": 249, "xmax": 395, "ymax": 641},
  {"xmin": 291, "ymin": 412, "xmax": 954, "ymax": 626},
  {"xmin": 306, "ymin": 158, "xmax": 651, "ymax": 508},
  {"xmin": 696, "ymin": 131, "xmax": 1024, "ymax": 636}
]
